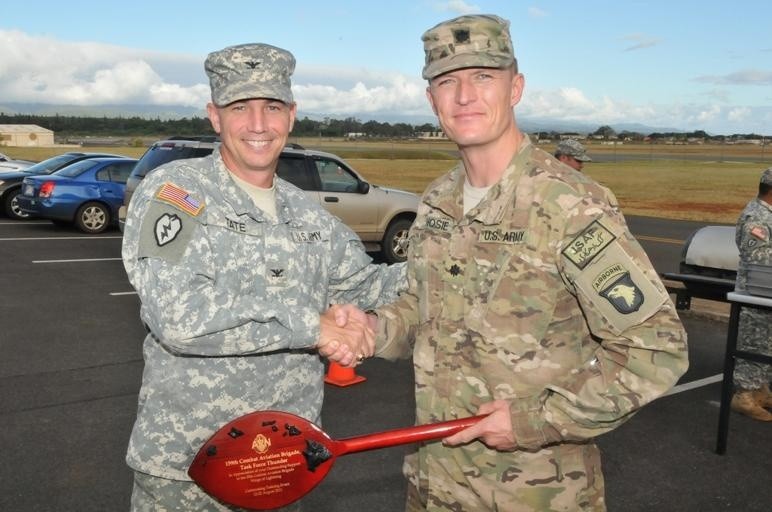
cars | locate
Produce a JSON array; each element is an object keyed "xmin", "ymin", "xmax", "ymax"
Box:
[{"xmin": 0, "ymin": 151, "xmax": 140, "ymax": 235}]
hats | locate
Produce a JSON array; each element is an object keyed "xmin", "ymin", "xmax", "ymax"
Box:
[
  {"xmin": 555, "ymin": 139, "xmax": 592, "ymax": 162},
  {"xmin": 760, "ymin": 167, "xmax": 772, "ymax": 186},
  {"xmin": 420, "ymin": 13, "xmax": 515, "ymax": 80},
  {"xmin": 205, "ymin": 43, "xmax": 295, "ymax": 107}
]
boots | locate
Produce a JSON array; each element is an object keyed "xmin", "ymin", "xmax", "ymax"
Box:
[
  {"xmin": 753, "ymin": 383, "xmax": 771, "ymax": 408},
  {"xmin": 730, "ymin": 386, "xmax": 771, "ymax": 422}
]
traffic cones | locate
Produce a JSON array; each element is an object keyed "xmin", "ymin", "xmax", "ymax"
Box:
[{"xmin": 322, "ymin": 362, "xmax": 367, "ymax": 388}]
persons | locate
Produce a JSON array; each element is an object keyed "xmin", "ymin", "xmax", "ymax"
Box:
[
  {"xmin": 554, "ymin": 139, "xmax": 592, "ymax": 172},
  {"xmin": 729, "ymin": 166, "xmax": 772, "ymax": 422},
  {"xmin": 120, "ymin": 42, "xmax": 410, "ymax": 512},
  {"xmin": 316, "ymin": 12, "xmax": 691, "ymax": 511}
]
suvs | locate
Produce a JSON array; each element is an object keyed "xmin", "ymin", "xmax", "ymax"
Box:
[{"xmin": 115, "ymin": 132, "xmax": 426, "ymax": 267}]
